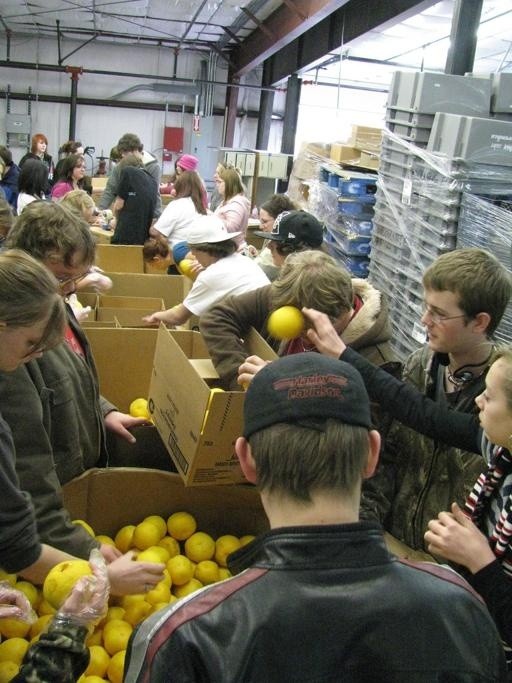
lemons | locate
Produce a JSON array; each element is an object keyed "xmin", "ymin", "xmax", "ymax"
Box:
[
  {"xmin": 267, "ymin": 307, "xmax": 306, "ymax": 340},
  {"xmin": 129, "ymin": 398, "xmax": 151, "ymax": 421},
  {"xmin": 0, "ymin": 511, "xmax": 256, "ymax": 682}
]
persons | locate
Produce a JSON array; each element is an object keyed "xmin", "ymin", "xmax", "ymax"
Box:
[
  {"xmin": 1, "ymin": 198, "xmax": 176, "ymax": 484},
  {"xmin": 149, "ymin": 155, "xmax": 259, "ymax": 279},
  {"xmin": 360, "ymin": 247, "xmax": 512, "ymax": 555},
  {"xmin": 96, "ymin": 134, "xmax": 161, "ymax": 245},
  {"xmin": 239, "ymin": 194, "xmax": 322, "ymax": 283},
  {"xmin": 120, "ymin": 349, "xmax": 503, "ymax": 682},
  {"xmin": 1, "ymin": 135, "xmax": 113, "ymax": 324},
  {"xmin": 142, "ymin": 216, "xmax": 271, "ymax": 325},
  {"xmin": 1, "ymin": 248, "xmax": 164, "ymax": 598},
  {"xmin": 300, "ymin": 308, "xmax": 512, "ymax": 667},
  {"xmin": 0, "ymin": 548, "xmax": 111, "ymax": 683},
  {"xmin": 201, "ymin": 249, "xmax": 402, "ymax": 393}
]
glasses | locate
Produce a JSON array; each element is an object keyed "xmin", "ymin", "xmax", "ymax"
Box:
[
  {"xmin": 421, "ymin": 302, "xmax": 465, "ymax": 325},
  {"xmin": 55, "ymin": 270, "xmax": 90, "ymax": 290},
  {"xmin": 216, "ymin": 180, "xmax": 224, "ymax": 183}
]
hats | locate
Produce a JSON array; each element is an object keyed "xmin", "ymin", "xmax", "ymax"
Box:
[
  {"xmin": 243, "ymin": 351, "xmax": 373, "ymax": 443},
  {"xmin": 176, "ymin": 154, "xmax": 200, "ymax": 172},
  {"xmin": 186, "ymin": 216, "xmax": 243, "ymax": 245},
  {"xmin": 253, "ymin": 209, "xmax": 323, "ymax": 248}
]
